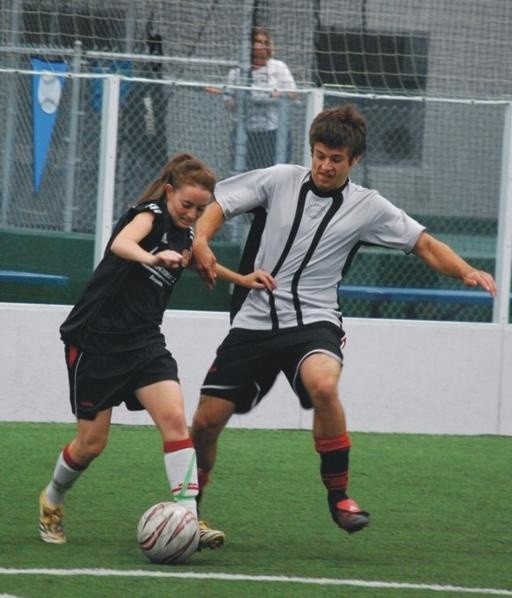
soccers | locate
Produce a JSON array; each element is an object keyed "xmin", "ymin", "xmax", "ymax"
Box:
[{"xmin": 137, "ymin": 502, "xmax": 200, "ymax": 564}]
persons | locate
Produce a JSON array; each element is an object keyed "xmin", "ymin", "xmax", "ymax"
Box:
[
  {"xmin": 188, "ymin": 104, "xmax": 501, "ymax": 536},
  {"xmin": 219, "ymin": 28, "xmax": 299, "ymax": 175},
  {"xmin": 33, "ymin": 151, "xmax": 278, "ymax": 555},
  {"xmin": 367, "ymin": 124, "xmax": 435, "ymax": 320}
]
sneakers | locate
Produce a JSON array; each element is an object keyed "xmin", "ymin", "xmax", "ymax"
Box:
[
  {"xmin": 196, "ymin": 520, "xmax": 227, "ymax": 553},
  {"xmin": 38, "ymin": 489, "xmax": 68, "ymax": 546},
  {"xmin": 326, "ymin": 493, "xmax": 370, "ymax": 535}
]
